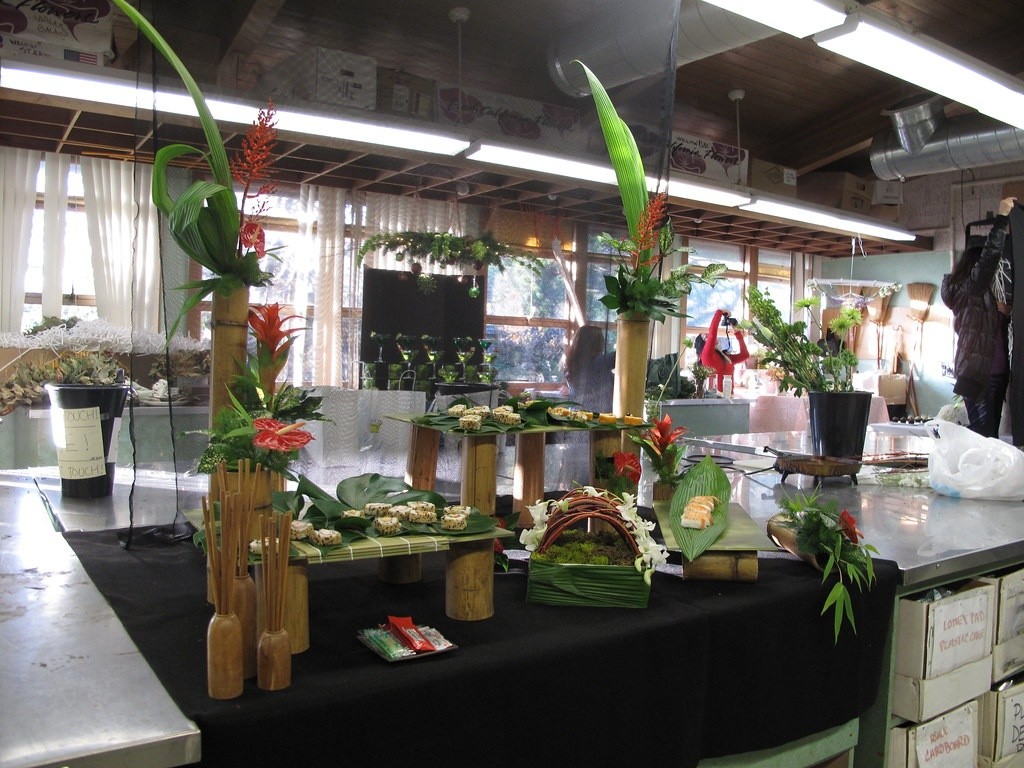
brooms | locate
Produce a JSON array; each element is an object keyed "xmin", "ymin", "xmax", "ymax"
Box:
[
  {"xmin": 905, "ymin": 284, "xmax": 937, "ymax": 353},
  {"xmin": 838, "ymin": 286, "xmax": 893, "ymax": 369}
]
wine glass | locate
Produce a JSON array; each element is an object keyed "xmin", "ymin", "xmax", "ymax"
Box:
[{"xmin": 373, "ymin": 333, "xmax": 499, "ymax": 384}]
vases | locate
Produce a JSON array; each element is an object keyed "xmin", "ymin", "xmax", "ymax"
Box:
[
  {"xmin": 807, "ymin": 389, "xmax": 873, "ymax": 488},
  {"xmin": 45, "ymin": 382, "xmax": 133, "ymax": 498},
  {"xmin": 697, "ymin": 380, "xmax": 706, "ymax": 399},
  {"xmin": 722, "ymin": 375, "xmax": 732, "ymax": 399}
]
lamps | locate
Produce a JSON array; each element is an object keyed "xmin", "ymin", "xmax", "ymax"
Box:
[
  {"xmin": 739, "ymin": 194, "xmax": 917, "ymax": 242},
  {"xmin": 0, "ymin": 43, "xmax": 474, "ymax": 158},
  {"xmin": 464, "ymin": 135, "xmax": 751, "ymax": 209}
]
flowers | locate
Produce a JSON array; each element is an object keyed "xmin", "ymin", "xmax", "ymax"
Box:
[
  {"xmin": 774, "ymin": 483, "xmax": 878, "ymax": 646},
  {"xmin": 643, "ymin": 381, "xmax": 674, "ymax": 401},
  {"xmin": 737, "ymin": 282, "xmax": 864, "ymax": 396},
  {"xmin": 573, "ymin": 58, "xmax": 728, "ymax": 325},
  {"xmin": 112, "ymin": 0, "xmax": 287, "ymax": 341},
  {"xmin": 515, "ymin": 482, "xmax": 670, "ymax": 588},
  {"xmin": 630, "ymin": 411, "xmax": 689, "ymax": 485},
  {"xmin": 205, "ymin": 296, "xmax": 328, "ymax": 466},
  {"xmin": 687, "ymin": 360, "xmax": 716, "ymax": 383},
  {"xmin": 591, "ymin": 448, "xmax": 643, "ymax": 491}
]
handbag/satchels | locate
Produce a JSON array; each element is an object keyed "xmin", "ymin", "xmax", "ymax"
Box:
[{"xmin": 923, "ymin": 419, "xmax": 1024, "ymax": 502}]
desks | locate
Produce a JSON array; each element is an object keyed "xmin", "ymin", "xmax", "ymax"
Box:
[
  {"xmin": 29, "ymin": 415, "xmax": 1024, "ymax": 768},
  {"xmin": 869, "ymin": 421, "xmax": 939, "ymax": 438},
  {"xmin": 0, "ymin": 474, "xmax": 202, "ymax": 768}
]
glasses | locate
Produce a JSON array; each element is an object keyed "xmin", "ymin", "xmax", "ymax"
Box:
[{"xmin": 704, "ymin": 333, "xmax": 709, "ymax": 341}]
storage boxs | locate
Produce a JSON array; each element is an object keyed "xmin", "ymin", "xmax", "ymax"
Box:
[
  {"xmin": 747, "ymin": 158, "xmax": 797, "ymax": 199},
  {"xmin": 377, "ymin": 66, "xmax": 438, "ymax": 124},
  {"xmin": 887, "ymin": 566, "xmax": 1024, "ymax": 768},
  {"xmin": 252, "ymin": 45, "xmax": 379, "ymax": 114},
  {"xmin": 121, "ymin": 22, "xmax": 223, "ymax": 86},
  {"xmin": 0, "ymin": 0, "xmax": 115, "ymax": 68},
  {"xmin": 578, "ymin": 111, "xmax": 749, "ymax": 186},
  {"xmin": 796, "ymin": 171, "xmax": 907, "ymax": 225},
  {"xmin": 435, "ymin": 78, "xmax": 580, "ymax": 158}
]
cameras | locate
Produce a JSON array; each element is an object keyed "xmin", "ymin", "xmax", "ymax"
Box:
[{"xmin": 721, "ymin": 313, "xmax": 737, "ymax": 326}]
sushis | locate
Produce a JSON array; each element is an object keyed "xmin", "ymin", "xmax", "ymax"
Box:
[
  {"xmin": 250, "ymin": 501, "xmax": 471, "ymax": 554},
  {"xmin": 447, "ymin": 400, "xmax": 642, "ymax": 430},
  {"xmin": 680, "ymin": 496, "xmax": 719, "ymax": 529}
]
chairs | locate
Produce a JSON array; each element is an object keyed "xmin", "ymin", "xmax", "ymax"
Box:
[
  {"xmin": 700, "ymin": 717, "xmax": 861, "ymax": 768},
  {"xmin": 748, "ymin": 396, "xmax": 809, "ymax": 433},
  {"xmin": 878, "ymin": 374, "xmax": 906, "ymax": 422},
  {"xmin": 867, "ymin": 396, "xmax": 890, "ymax": 424}
]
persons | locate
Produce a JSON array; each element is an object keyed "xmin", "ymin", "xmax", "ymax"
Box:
[
  {"xmin": 562, "ymin": 325, "xmax": 618, "ymax": 414},
  {"xmin": 694, "ymin": 308, "xmax": 749, "ymax": 397},
  {"xmin": 940, "ymin": 197, "xmax": 1017, "ymax": 440}
]
